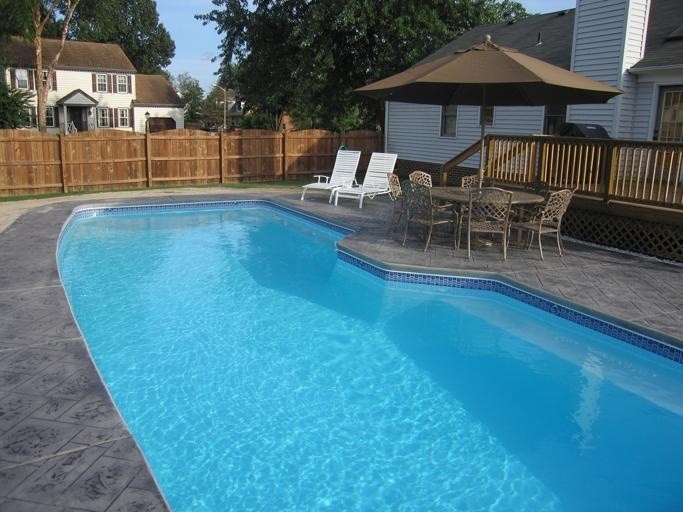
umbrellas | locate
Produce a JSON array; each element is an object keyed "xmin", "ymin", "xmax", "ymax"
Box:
[{"xmin": 352, "ymin": 35, "xmax": 626, "ymax": 187}]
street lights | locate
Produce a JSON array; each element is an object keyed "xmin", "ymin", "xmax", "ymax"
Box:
[
  {"xmin": 210, "ymin": 83, "xmax": 226, "ymax": 130},
  {"xmin": 144, "ymin": 111, "xmax": 150, "ymax": 131}
]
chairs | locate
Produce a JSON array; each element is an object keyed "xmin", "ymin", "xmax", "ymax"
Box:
[{"xmin": 302, "ymin": 147, "xmax": 572, "ymax": 261}]
